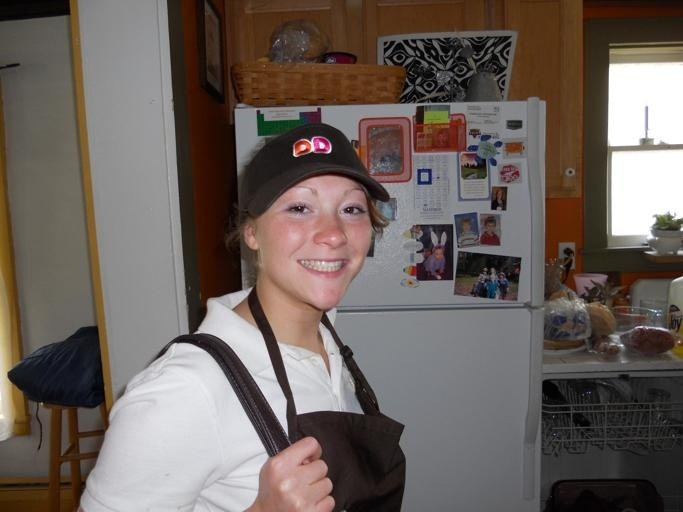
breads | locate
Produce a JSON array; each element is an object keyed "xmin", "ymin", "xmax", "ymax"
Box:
[
  {"xmin": 587, "ymin": 303, "xmax": 616, "ymax": 336},
  {"xmin": 620, "ymin": 326, "xmax": 675, "ymax": 354}
]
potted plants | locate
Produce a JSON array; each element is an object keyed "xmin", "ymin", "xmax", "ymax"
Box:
[{"xmin": 652, "ymin": 215, "xmax": 683, "ymax": 255}]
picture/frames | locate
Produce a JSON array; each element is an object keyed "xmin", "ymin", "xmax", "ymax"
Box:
[{"xmin": 195, "ymin": 0, "xmax": 225, "ymax": 105}]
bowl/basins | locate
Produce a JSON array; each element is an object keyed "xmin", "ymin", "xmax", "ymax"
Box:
[{"xmin": 610, "ymin": 305, "xmax": 658, "ymax": 337}]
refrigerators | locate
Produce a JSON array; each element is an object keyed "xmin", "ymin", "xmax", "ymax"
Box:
[{"xmin": 232, "ymin": 96, "xmax": 550, "ymax": 512}]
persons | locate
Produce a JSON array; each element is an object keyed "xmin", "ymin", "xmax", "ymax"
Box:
[
  {"xmin": 472, "ymin": 265, "xmax": 509, "ymax": 298},
  {"xmin": 458, "ymin": 218, "xmax": 477, "ymax": 247},
  {"xmin": 478, "ymin": 215, "xmax": 497, "ymax": 238},
  {"xmin": 71, "ymin": 122, "xmax": 405, "ymax": 510},
  {"xmin": 419, "ymin": 244, "xmax": 447, "ymax": 281},
  {"xmin": 493, "ymin": 188, "xmax": 504, "ymax": 209}
]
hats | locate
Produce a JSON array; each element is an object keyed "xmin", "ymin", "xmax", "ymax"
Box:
[{"xmin": 241, "ymin": 121, "xmax": 389, "ymax": 218}]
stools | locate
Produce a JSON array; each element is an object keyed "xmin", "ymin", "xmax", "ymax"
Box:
[{"xmin": 41, "ymin": 399, "xmax": 110, "ymax": 512}]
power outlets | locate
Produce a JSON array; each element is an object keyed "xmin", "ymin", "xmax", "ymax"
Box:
[{"xmin": 558, "ymin": 242, "xmax": 576, "ymax": 269}]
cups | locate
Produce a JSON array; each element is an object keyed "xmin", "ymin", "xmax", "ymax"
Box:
[
  {"xmin": 645, "ymin": 386, "xmax": 673, "ymax": 421},
  {"xmin": 639, "ymin": 297, "xmax": 668, "ymax": 330}
]
